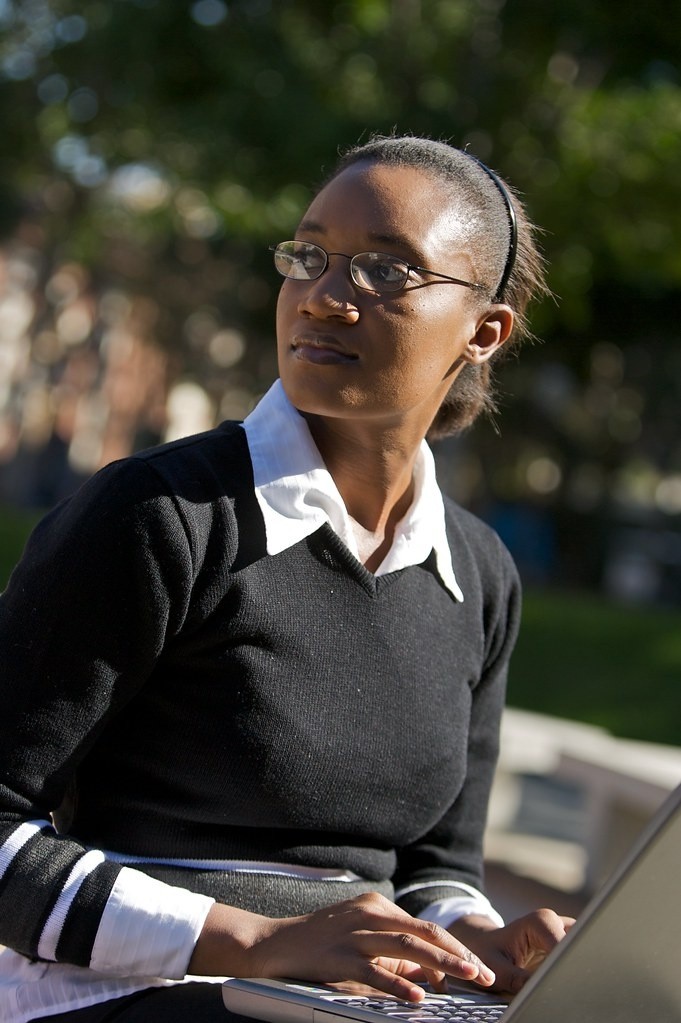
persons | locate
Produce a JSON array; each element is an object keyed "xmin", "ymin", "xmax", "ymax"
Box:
[{"xmin": 0, "ymin": 137, "xmax": 576, "ymax": 1022}]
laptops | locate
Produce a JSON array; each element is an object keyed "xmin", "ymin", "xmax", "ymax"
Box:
[{"xmin": 220, "ymin": 779, "xmax": 680, "ymax": 1023}]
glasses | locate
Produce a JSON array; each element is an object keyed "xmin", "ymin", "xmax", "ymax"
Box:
[{"xmin": 268, "ymin": 240, "xmax": 487, "ymax": 294}]
